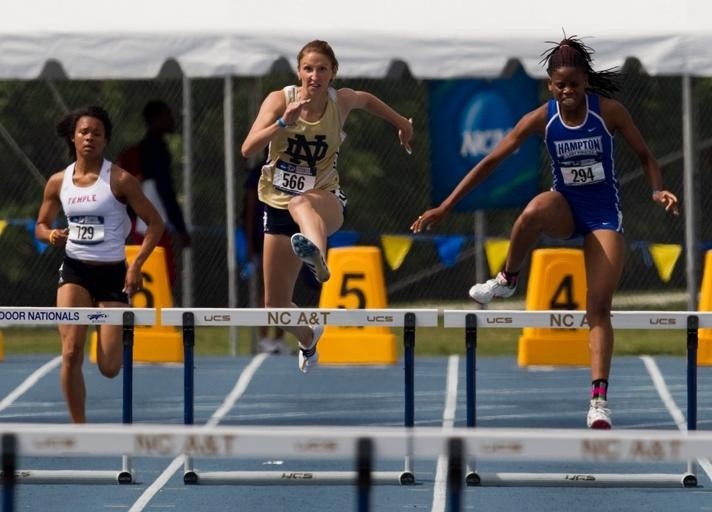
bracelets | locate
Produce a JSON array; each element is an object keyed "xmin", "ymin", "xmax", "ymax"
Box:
[
  {"xmin": 277, "ymin": 117, "xmax": 287, "ymax": 129},
  {"xmin": 646, "ymin": 188, "xmax": 663, "ymax": 198},
  {"xmin": 50, "ymin": 229, "xmax": 56, "ymax": 243}
]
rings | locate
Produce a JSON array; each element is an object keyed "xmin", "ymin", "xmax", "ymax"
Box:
[{"xmin": 418, "ymin": 216, "xmax": 422, "ymax": 219}]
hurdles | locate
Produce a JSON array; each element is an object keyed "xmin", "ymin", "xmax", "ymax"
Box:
[
  {"xmin": 161, "ymin": 307, "xmax": 439, "ymax": 487},
  {"xmin": 1, "ymin": 306, "xmax": 157, "ymax": 485},
  {"xmin": 3, "ymin": 421, "xmax": 440, "ymax": 512},
  {"xmin": 441, "ymin": 428, "xmax": 712, "ymax": 510},
  {"xmin": 442, "ymin": 307, "xmax": 711, "ymax": 489}
]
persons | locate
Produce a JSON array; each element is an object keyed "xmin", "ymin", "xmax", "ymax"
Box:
[
  {"xmin": 111, "ymin": 99, "xmax": 195, "ymax": 290},
  {"xmin": 408, "ymin": 27, "xmax": 681, "ymax": 431},
  {"xmin": 240, "ymin": 39, "xmax": 416, "ymax": 375},
  {"xmin": 32, "ymin": 104, "xmax": 168, "ymax": 426}
]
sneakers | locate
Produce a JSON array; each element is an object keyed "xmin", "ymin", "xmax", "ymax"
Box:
[
  {"xmin": 298, "ymin": 327, "xmax": 325, "ymax": 374},
  {"xmin": 468, "ymin": 269, "xmax": 518, "ymax": 305},
  {"xmin": 586, "ymin": 397, "xmax": 612, "ymax": 428},
  {"xmin": 291, "ymin": 232, "xmax": 330, "ymax": 283}
]
